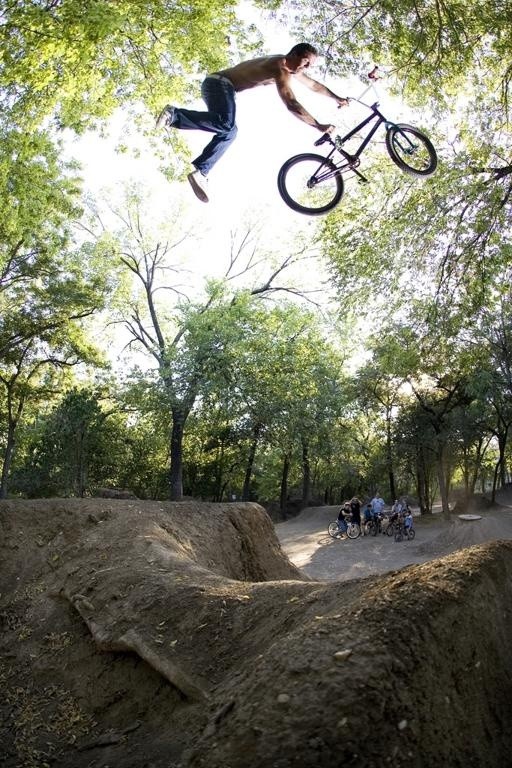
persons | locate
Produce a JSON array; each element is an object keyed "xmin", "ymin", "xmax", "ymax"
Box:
[
  {"xmin": 333, "ymin": 492, "xmax": 414, "ymax": 541},
  {"xmin": 155, "ymin": 43, "xmax": 350, "ymax": 203}
]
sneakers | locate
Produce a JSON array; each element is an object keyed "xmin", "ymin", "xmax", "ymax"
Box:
[
  {"xmin": 187, "ymin": 171, "xmax": 208, "ymax": 202},
  {"xmin": 155, "ymin": 105, "xmax": 174, "ymax": 130}
]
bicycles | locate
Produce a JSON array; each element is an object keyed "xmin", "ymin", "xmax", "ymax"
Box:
[
  {"xmin": 328, "ymin": 512, "xmax": 361, "ymax": 540},
  {"xmin": 278, "ymin": 66, "xmax": 436, "ymax": 216},
  {"xmin": 386, "ymin": 517, "xmax": 409, "ymax": 538},
  {"xmin": 394, "ymin": 520, "xmax": 416, "ymax": 542},
  {"xmin": 361, "ymin": 512, "xmax": 387, "ymax": 537}
]
